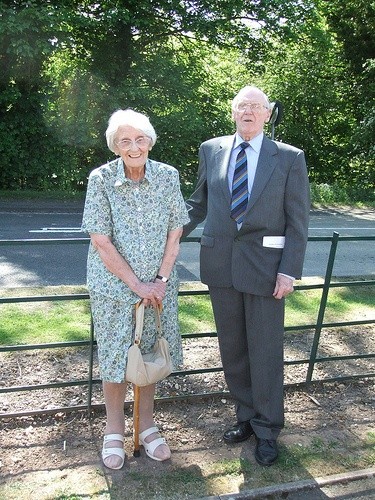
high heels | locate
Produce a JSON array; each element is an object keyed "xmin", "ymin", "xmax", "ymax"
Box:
[
  {"xmin": 133, "ymin": 426, "xmax": 171, "ymax": 461},
  {"xmin": 103, "ymin": 434, "xmax": 124, "ymax": 469}
]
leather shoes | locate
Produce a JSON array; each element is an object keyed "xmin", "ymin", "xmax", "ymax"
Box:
[
  {"xmin": 223, "ymin": 419, "xmax": 254, "ymax": 443},
  {"xmin": 255, "ymin": 433, "xmax": 278, "ymax": 467}
]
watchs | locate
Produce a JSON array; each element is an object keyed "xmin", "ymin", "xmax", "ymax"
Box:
[{"xmin": 156, "ymin": 274, "xmax": 170, "ymax": 284}]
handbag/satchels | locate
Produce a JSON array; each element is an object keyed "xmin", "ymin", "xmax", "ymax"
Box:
[{"xmin": 126, "ymin": 336, "xmax": 172, "ymax": 387}]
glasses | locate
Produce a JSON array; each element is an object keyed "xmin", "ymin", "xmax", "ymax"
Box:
[
  {"xmin": 114, "ymin": 137, "xmax": 151, "ymax": 151},
  {"xmin": 234, "ymin": 101, "xmax": 268, "ymax": 113}
]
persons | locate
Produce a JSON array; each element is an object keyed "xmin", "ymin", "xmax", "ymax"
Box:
[
  {"xmin": 80, "ymin": 107, "xmax": 192, "ymax": 470},
  {"xmin": 182, "ymin": 84, "xmax": 310, "ymax": 468}
]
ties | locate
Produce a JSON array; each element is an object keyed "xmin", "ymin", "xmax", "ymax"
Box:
[{"xmin": 230, "ymin": 142, "xmax": 250, "ymax": 224}]
